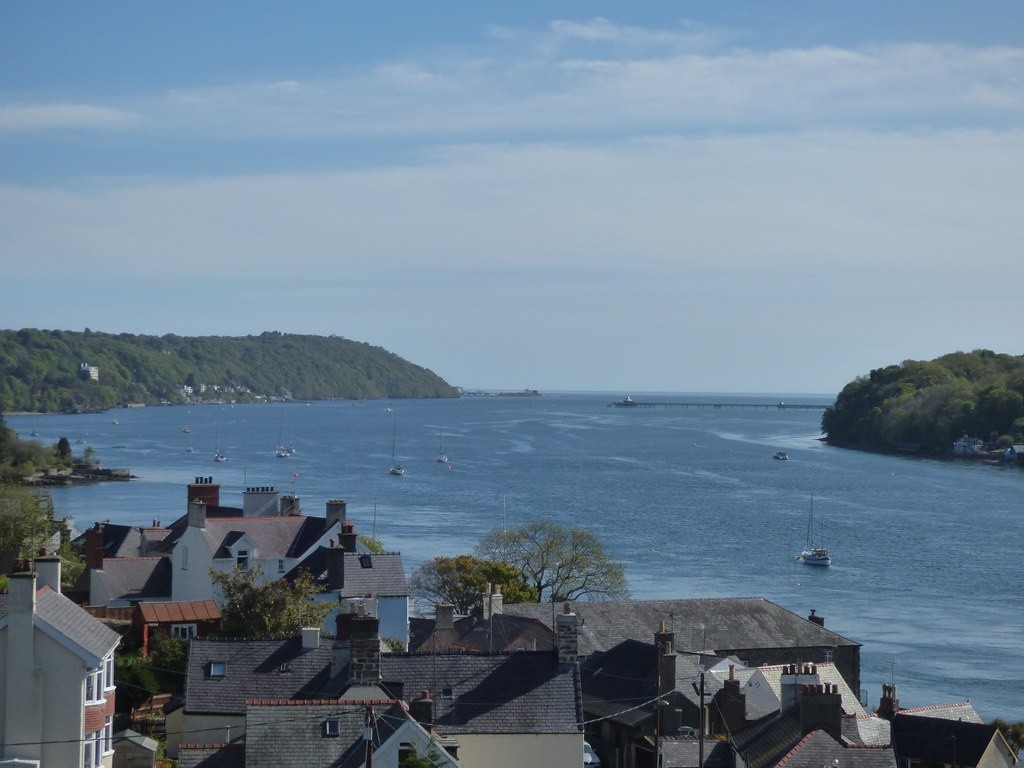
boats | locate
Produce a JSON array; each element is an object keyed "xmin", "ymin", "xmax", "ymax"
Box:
[
  {"xmin": 113, "ymin": 419, "xmax": 119, "ymax": 424},
  {"xmin": 32, "ymin": 431, "xmax": 39, "ymax": 436},
  {"xmin": 186, "ymin": 447, "xmax": 193, "ymax": 451},
  {"xmin": 77, "ymin": 438, "xmax": 85, "ymax": 443},
  {"xmin": 182, "ymin": 424, "xmax": 192, "ymax": 433},
  {"xmin": 385, "ymin": 408, "xmax": 392, "ymax": 412},
  {"xmin": 773, "ymin": 451, "xmax": 789, "ymax": 459}
]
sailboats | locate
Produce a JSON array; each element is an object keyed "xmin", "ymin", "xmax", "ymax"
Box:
[
  {"xmin": 390, "ymin": 418, "xmax": 404, "ymax": 475},
  {"xmin": 802, "ymin": 492, "xmax": 831, "ymax": 565},
  {"xmin": 275, "ymin": 408, "xmax": 295, "ymax": 458},
  {"xmin": 437, "ymin": 426, "xmax": 448, "ymax": 462},
  {"xmin": 214, "ymin": 421, "xmax": 227, "ymax": 461}
]
